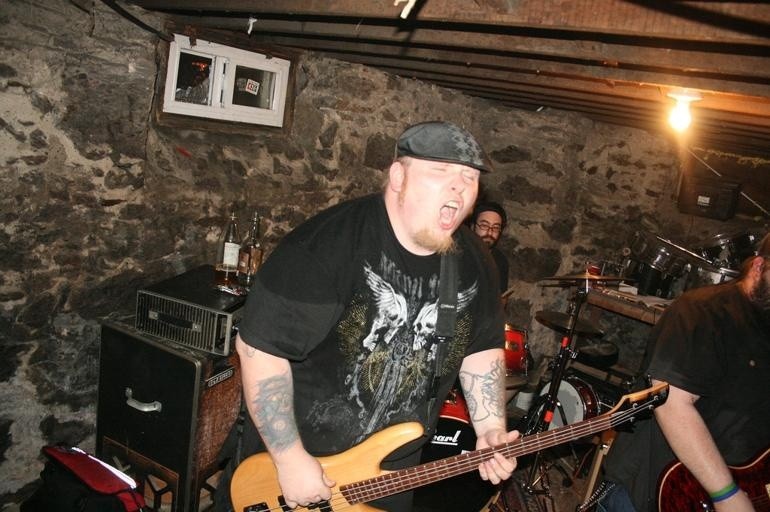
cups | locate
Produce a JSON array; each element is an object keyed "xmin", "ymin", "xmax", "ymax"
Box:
[
  {"xmin": 215, "ymin": 262, "xmax": 237, "ymax": 288},
  {"xmin": 588, "ymin": 264, "xmax": 600, "ymax": 276}
]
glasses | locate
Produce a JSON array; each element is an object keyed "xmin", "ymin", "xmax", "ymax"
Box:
[{"xmin": 473, "ymin": 222, "xmax": 502, "ymax": 232}]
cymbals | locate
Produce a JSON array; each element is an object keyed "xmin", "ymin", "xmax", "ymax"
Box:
[
  {"xmin": 535, "ymin": 310, "xmax": 608, "ymax": 335},
  {"xmin": 544, "ymin": 273, "xmax": 635, "ymax": 282}
]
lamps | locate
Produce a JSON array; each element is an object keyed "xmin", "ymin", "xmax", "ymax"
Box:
[{"xmin": 664, "ymin": 89, "xmax": 703, "ymax": 130}]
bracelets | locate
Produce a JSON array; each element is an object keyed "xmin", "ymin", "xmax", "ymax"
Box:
[{"xmin": 709, "ymin": 481, "xmax": 739, "ymax": 503}]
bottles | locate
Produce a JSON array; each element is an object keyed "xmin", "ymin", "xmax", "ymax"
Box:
[
  {"xmin": 217, "ymin": 210, "xmax": 241, "ymax": 267},
  {"xmin": 237, "ymin": 217, "xmax": 264, "ymax": 287}
]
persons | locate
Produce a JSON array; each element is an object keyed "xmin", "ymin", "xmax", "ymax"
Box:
[
  {"xmin": 469, "ymin": 200, "xmax": 510, "ymax": 311},
  {"xmin": 588, "ymin": 231, "xmax": 770, "ymax": 512},
  {"xmin": 213, "ymin": 117, "xmax": 521, "ymax": 511}
]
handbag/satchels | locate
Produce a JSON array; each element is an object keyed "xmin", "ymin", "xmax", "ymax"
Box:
[{"xmin": 19, "ymin": 446, "xmax": 144, "ymax": 510}]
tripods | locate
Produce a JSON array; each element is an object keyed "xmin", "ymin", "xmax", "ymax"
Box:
[{"xmin": 510, "ymin": 343, "xmax": 580, "ymax": 499}]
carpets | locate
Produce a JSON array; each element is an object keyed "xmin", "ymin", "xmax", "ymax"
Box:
[{"xmin": 489, "ymin": 465, "xmax": 545, "ymax": 512}]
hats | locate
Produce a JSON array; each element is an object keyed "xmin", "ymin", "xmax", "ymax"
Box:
[
  {"xmin": 472, "ymin": 202, "xmax": 506, "ymax": 225},
  {"xmin": 393, "ymin": 121, "xmax": 495, "ymax": 175}
]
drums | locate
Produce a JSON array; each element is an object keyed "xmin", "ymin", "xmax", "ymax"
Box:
[
  {"xmin": 537, "ymin": 378, "xmax": 599, "ymax": 431},
  {"xmin": 647, "ymin": 245, "xmax": 687, "ymax": 278},
  {"xmin": 504, "ymin": 324, "xmax": 531, "ymax": 376},
  {"xmin": 696, "ymin": 264, "xmax": 741, "ymax": 287},
  {"xmin": 698, "ymin": 230, "xmax": 761, "ymax": 269},
  {"xmin": 438, "ymin": 387, "xmax": 473, "ymax": 426}
]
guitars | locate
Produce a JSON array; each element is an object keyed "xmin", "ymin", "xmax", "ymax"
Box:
[
  {"xmin": 656, "ymin": 446, "xmax": 770, "ymax": 510},
  {"xmin": 230, "ymin": 380, "xmax": 670, "ymax": 512}
]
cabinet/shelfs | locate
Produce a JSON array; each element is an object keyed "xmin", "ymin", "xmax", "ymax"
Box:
[{"xmin": 95, "ymin": 312, "xmax": 244, "ymax": 512}]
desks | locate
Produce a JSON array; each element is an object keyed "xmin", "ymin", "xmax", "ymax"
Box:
[{"xmin": 568, "ymin": 289, "xmax": 669, "ymax": 403}]
